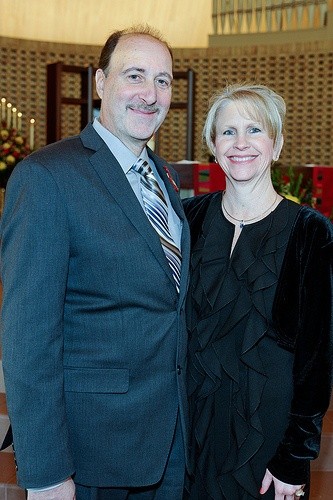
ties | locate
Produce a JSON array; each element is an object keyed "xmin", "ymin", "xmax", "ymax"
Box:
[{"xmin": 132, "ymin": 158, "xmax": 181, "ymax": 294}]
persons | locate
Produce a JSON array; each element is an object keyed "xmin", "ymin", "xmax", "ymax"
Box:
[
  {"xmin": 0, "ymin": 24, "xmax": 197, "ymax": 500},
  {"xmin": 182, "ymin": 82, "xmax": 333, "ymax": 500}
]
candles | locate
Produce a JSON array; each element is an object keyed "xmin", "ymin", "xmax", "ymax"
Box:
[
  {"xmin": 30, "ymin": 118, "xmax": 35, "ymax": 151},
  {"xmin": 7, "ymin": 103, "xmax": 12, "ymax": 129},
  {"xmin": 12, "ymin": 108, "xmax": 17, "ymax": 128},
  {"xmin": 17, "ymin": 112, "xmax": 22, "ymax": 131},
  {"xmin": 1, "ymin": 98, "xmax": 6, "ymax": 122}
]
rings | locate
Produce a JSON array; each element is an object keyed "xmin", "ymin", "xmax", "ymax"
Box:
[{"xmin": 295, "ymin": 490, "xmax": 305, "ymax": 497}]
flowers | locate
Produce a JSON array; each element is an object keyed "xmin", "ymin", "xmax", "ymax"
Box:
[
  {"xmin": 0, "ymin": 120, "xmax": 32, "ymax": 171},
  {"xmin": 272, "ymin": 168, "xmax": 317, "ymax": 211}
]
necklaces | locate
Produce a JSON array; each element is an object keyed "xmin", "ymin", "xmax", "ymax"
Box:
[{"xmin": 222, "ymin": 189, "xmax": 277, "ymax": 228}]
